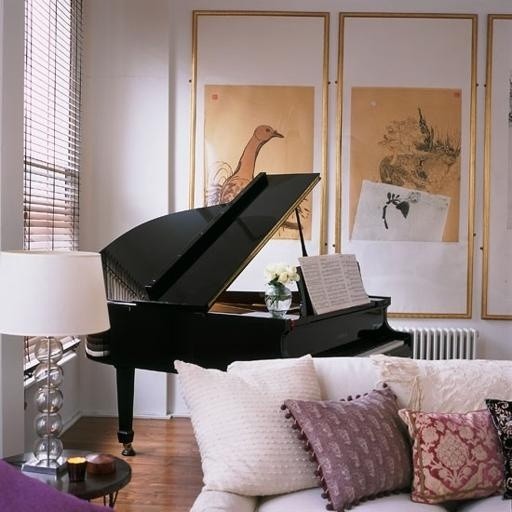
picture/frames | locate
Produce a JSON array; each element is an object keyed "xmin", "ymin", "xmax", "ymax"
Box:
[
  {"xmin": 190, "ymin": 10, "xmax": 330, "ymax": 294},
  {"xmin": 480, "ymin": 14, "xmax": 510, "ymax": 322},
  {"xmin": 335, "ymin": 10, "xmax": 480, "ymax": 320}
]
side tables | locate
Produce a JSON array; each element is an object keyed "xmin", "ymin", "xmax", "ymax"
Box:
[{"xmin": 1, "ymin": 447, "xmax": 132, "ymax": 510}]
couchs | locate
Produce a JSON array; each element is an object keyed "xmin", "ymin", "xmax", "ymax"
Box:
[{"xmin": 186, "ymin": 358, "xmax": 511, "ymax": 512}]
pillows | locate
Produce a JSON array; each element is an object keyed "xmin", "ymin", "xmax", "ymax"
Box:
[
  {"xmin": 482, "ymin": 398, "xmax": 510, "ymax": 503},
  {"xmin": 279, "ymin": 383, "xmax": 415, "ymax": 510},
  {"xmin": 171, "ymin": 359, "xmax": 328, "ymax": 496},
  {"xmin": 395, "ymin": 405, "xmax": 504, "ymax": 510}
]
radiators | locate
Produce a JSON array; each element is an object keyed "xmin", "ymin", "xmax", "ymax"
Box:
[{"xmin": 388, "ymin": 328, "xmax": 483, "ymax": 360}]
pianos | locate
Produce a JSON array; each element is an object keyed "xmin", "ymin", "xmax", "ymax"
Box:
[{"xmin": 85, "ymin": 172, "xmax": 412, "ymax": 457}]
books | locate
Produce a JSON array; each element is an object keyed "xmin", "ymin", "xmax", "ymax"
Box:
[{"xmin": 296, "ymin": 256, "xmax": 371, "ymax": 316}]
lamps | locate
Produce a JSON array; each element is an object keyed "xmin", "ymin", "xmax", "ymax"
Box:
[{"xmin": 1, "ymin": 250, "xmax": 113, "ymax": 476}]
